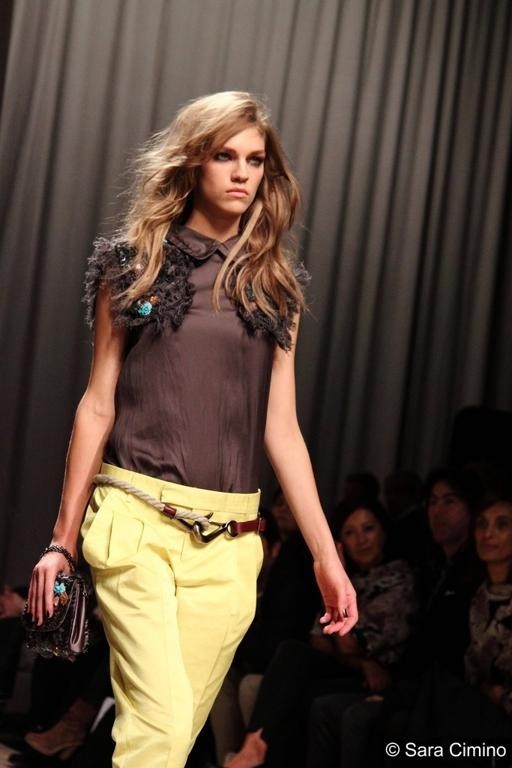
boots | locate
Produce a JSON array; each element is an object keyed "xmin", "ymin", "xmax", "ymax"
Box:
[{"xmin": 24, "ymin": 698, "xmax": 96, "ymax": 760}]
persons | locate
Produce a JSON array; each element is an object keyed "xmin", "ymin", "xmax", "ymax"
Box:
[{"xmin": 24, "ymin": 90, "xmax": 361, "ymax": 768}]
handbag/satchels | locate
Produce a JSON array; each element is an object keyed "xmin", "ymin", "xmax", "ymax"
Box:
[{"xmin": 23, "ymin": 546, "xmax": 90, "ymax": 662}]
